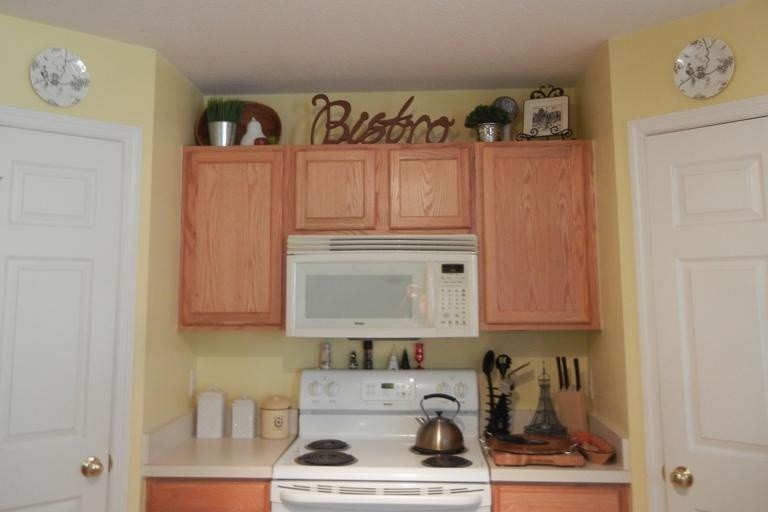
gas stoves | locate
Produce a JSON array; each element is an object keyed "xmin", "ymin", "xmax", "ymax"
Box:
[{"xmin": 272, "ymin": 421, "xmax": 492, "ymax": 483}]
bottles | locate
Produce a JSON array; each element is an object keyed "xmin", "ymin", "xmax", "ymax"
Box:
[
  {"xmin": 230, "ymin": 394, "xmax": 255, "ymax": 440},
  {"xmin": 400, "ymin": 349, "xmax": 411, "ymax": 369},
  {"xmin": 387, "ymin": 350, "xmax": 398, "ymax": 369},
  {"xmin": 195, "ymin": 386, "xmax": 226, "ymax": 440},
  {"xmin": 240, "ymin": 117, "xmax": 264, "ymax": 147},
  {"xmin": 257, "ymin": 393, "xmax": 293, "ymax": 439},
  {"xmin": 363, "ymin": 340, "xmax": 373, "ymax": 369},
  {"xmin": 320, "ymin": 342, "xmax": 332, "ymax": 369},
  {"xmin": 348, "ymin": 351, "xmax": 358, "ymax": 369}
]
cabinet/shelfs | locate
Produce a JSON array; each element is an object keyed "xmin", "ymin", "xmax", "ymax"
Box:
[
  {"xmin": 180, "ymin": 147, "xmax": 284, "ymax": 328},
  {"xmin": 477, "ymin": 141, "xmax": 601, "ymax": 336},
  {"xmin": 283, "ymin": 145, "xmax": 473, "ymax": 235}
]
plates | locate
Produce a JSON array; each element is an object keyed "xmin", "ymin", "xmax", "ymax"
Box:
[
  {"xmin": 669, "ymin": 35, "xmax": 742, "ymax": 100},
  {"xmin": 29, "ymin": 43, "xmax": 90, "ymax": 108},
  {"xmin": 492, "ymin": 96, "xmax": 520, "ymax": 125}
]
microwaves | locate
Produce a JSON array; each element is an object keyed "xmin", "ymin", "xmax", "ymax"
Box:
[{"xmin": 283, "ymin": 228, "xmax": 482, "ymax": 341}]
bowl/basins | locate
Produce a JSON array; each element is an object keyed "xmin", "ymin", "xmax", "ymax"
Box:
[{"xmin": 570, "ymin": 431, "xmax": 616, "ymax": 465}]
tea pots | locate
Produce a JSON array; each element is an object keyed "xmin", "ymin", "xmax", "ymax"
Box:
[{"xmin": 412, "ymin": 393, "xmax": 468, "ymax": 453}]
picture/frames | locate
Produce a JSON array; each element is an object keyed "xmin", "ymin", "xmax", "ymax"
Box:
[{"xmin": 521, "ymin": 96, "xmax": 569, "ymax": 136}]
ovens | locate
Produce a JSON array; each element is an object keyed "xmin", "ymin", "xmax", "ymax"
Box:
[{"xmin": 268, "ymin": 476, "xmax": 494, "ymax": 512}]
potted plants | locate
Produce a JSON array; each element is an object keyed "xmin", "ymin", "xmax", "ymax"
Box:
[
  {"xmin": 207, "ymin": 98, "xmax": 245, "ymax": 146},
  {"xmin": 465, "ymin": 105, "xmax": 512, "ymax": 140}
]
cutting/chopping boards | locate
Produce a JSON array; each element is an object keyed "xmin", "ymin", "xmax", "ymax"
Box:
[{"xmin": 485, "ymin": 435, "xmax": 586, "ymax": 468}]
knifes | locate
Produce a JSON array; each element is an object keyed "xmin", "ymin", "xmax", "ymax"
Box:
[
  {"xmin": 573, "ymin": 358, "xmax": 583, "ymax": 394},
  {"xmin": 556, "ymin": 356, "xmax": 564, "ymax": 392},
  {"xmin": 561, "ymin": 356, "xmax": 571, "ymax": 389}
]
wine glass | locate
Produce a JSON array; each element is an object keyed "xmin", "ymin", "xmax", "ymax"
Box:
[{"xmin": 415, "ymin": 342, "xmax": 424, "ymax": 369}]
